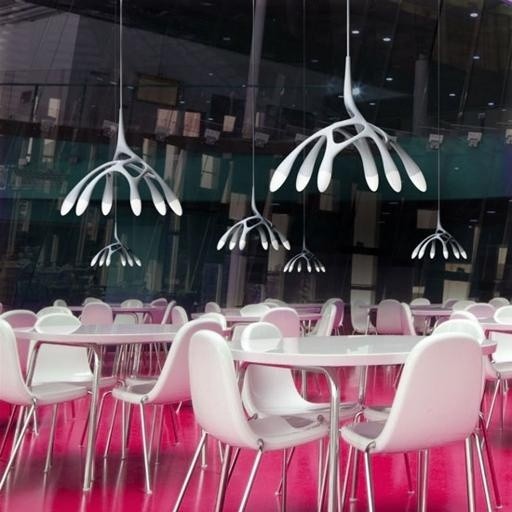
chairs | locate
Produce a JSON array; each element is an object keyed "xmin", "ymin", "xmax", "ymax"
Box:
[
  {"xmin": 0, "ymin": 319, "xmax": 96, "ymax": 492},
  {"xmin": 96, "ymin": 316, "xmax": 223, "ymax": 494},
  {"xmin": 450, "ymin": 312, "xmax": 511, "ymax": 445},
  {"xmin": 236, "ymin": 319, "xmax": 361, "ymax": 511},
  {"xmin": 0, "ymin": 295, "xmax": 512, "ymax": 373},
  {"xmin": 168, "ymin": 328, "xmax": 328, "ymax": 512},
  {"xmin": 24, "ymin": 313, "xmax": 128, "ymax": 464},
  {"xmin": 339, "ymin": 334, "xmax": 493, "ymax": 512}
]
